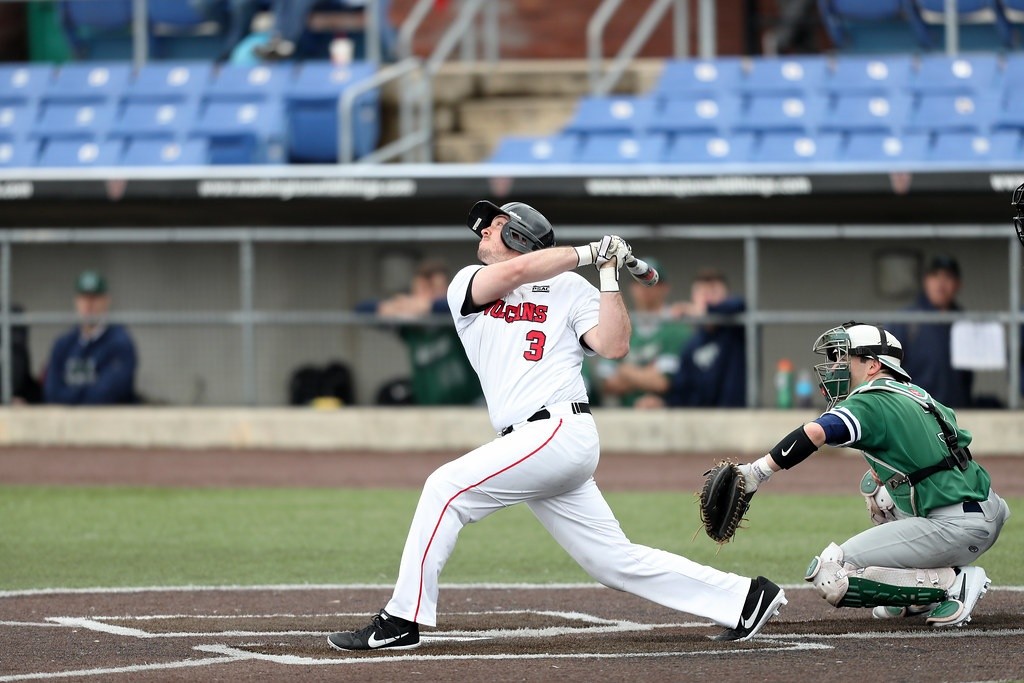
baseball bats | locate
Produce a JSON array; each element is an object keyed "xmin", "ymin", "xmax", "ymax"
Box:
[{"xmin": 606, "ymin": 241, "xmax": 660, "ymax": 287}]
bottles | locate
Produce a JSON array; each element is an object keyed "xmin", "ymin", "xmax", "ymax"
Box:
[
  {"xmin": 795, "ymin": 368, "xmax": 812, "ymax": 408},
  {"xmin": 772, "ymin": 358, "xmax": 793, "ymax": 408}
]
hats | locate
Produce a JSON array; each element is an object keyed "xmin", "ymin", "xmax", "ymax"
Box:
[{"xmin": 77, "ymin": 267, "xmax": 107, "ymax": 297}]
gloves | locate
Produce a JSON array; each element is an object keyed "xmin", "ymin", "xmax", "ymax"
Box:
[{"xmin": 573, "ymin": 234, "xmax": 631, "ymax": 292}]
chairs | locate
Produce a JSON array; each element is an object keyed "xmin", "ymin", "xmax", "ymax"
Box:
[
  {"xmin": 491, "ymin": 52, "xmax": 1024, "ymax": 166},
  {"xmin": 1, "ymin": 59, "xmax": 380, "ymax": 165}
]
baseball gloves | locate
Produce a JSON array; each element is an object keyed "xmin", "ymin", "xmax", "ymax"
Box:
[{"xmin": 691, "ymin": 456, "xmax": 749, "ymax": 561}]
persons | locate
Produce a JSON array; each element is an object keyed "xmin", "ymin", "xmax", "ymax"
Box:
[
  {"xmin": 44, "ymin": 270, "xmax": 137, "ymax": 405},
  {"xmin": 326, "ymin": 200, "xmax": 788, "ymax": 646},
  {"xmin": 354, "ymin": 255, "xmax": 483, "ymax": 405},
  {"xmin": 700, "ymin": 320, "xmax": 1010, "ymax": 627},
  {"xmin": 887, "ymin": 256, "xmax": 974, "ymax": 408},
  {"xmin": 595, "ymin": 268, "xmax": 745, "ymax": 408}
]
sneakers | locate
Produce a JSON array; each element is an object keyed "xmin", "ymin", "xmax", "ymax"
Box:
[
  {"xmin": 327, "ymin": 607, "xmax": 422, "ymax": 652},
  {"xmin": 872, "ymin": 598, "xmax": 930, "ymax": 619},
  {"xmin": 714, "ymin": 575, "xmax": 789, "ymax": 642},
  {"xmin": 925, "ymin": 565, "xmax": 990, "ymax": 628}
]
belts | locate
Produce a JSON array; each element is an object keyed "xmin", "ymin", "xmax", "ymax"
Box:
[
  {"xmin": 964, "ymin": 500, "xmax": 983, "ymax": 512},
  {"xmin": 502, "ymin": 401, "xmax": 592, "ymax": 437}
]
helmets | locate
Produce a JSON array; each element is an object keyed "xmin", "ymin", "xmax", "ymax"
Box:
[
  {"xmin": 828, "ymin": 320, "xmax": 912, "ymax": 380},
  {"xmin": 465, "ymin": 200, "xmax": 557, "ymax": 256}
]
